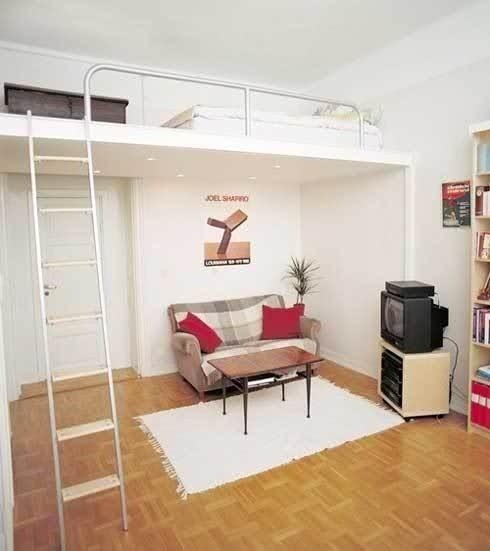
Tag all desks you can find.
[207,346,323,434]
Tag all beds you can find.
[159,105,385,151]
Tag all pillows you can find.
[177,313,223,354]
[259,304,304,340]
[316,101,378,123]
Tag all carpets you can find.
[130,376,408,502]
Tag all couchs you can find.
[166,292,325,401]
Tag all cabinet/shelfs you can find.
[4,80,131,125]
[377,343,453,423]
[464,116,490,441]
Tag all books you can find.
[471,141,490,428]
[248,372,277,387]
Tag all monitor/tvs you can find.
[380,290,448,354]
